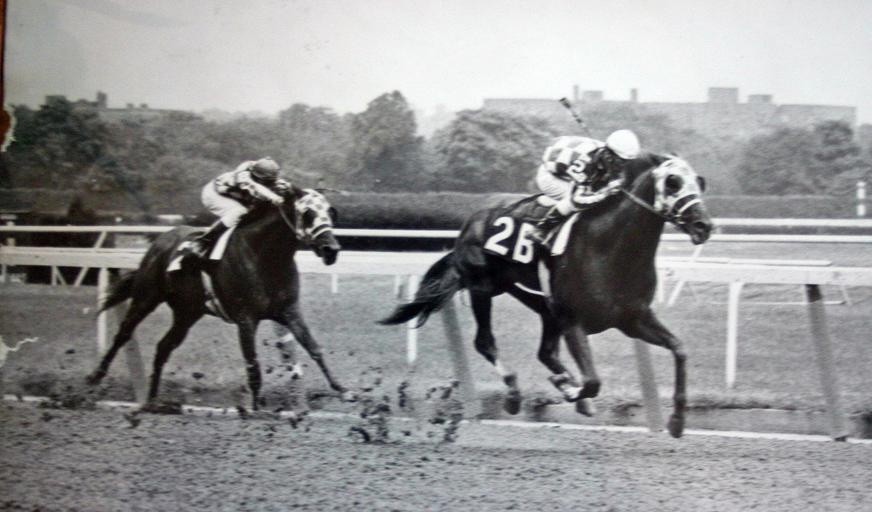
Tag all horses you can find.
[82,180,360,412]
[373,149,714,439]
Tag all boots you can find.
[525,207,569,248]
[193,221,228,257]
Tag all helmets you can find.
[605,129,641,160]
[251,156,279,181]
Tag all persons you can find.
[535,128,642,245]
[197,156,293,260]
